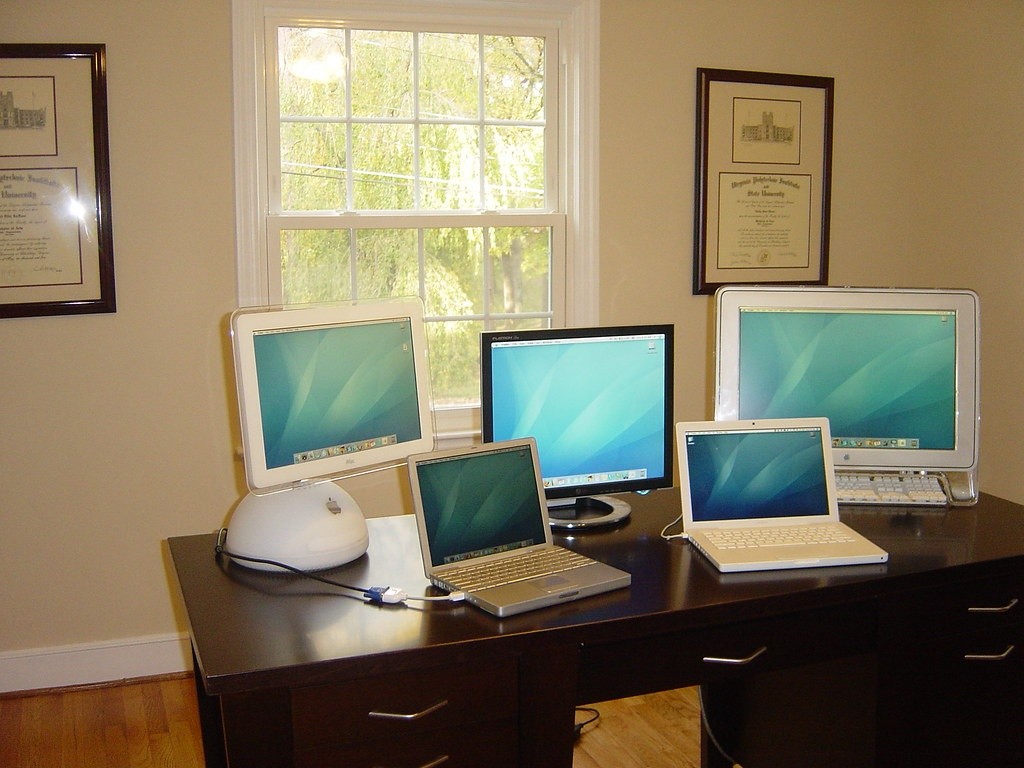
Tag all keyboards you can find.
[835,471,946,506]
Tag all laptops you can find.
[676,417,888,572]
[407,436,633,616]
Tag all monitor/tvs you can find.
[223,294,435,572]
[713,286,981,471]
[479,324,675,529]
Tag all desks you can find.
[167,485,1024,768]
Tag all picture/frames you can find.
[693,67,834,296]
[0,43,117,319]
